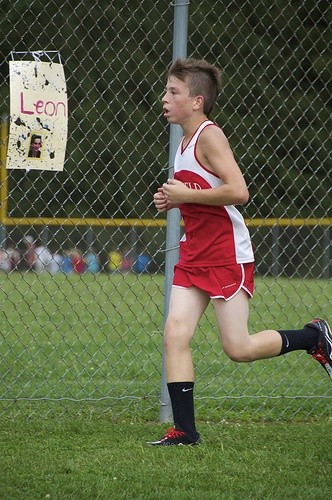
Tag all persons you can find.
[146,58,332,449]
[28,134,42,158]
[0,234,150,276]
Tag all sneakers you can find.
[305,318,332,380]
[147,428,200,447]
